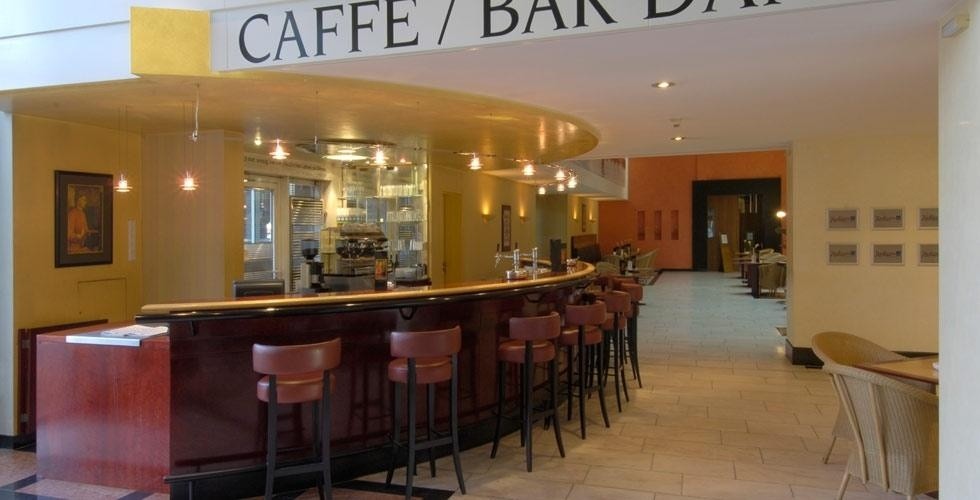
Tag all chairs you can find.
[490,275,646,475]
[249,336,352,498]
[379,324,469,499]
[734,245,787,298]
[810,329,941,498]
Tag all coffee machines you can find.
[300,238,331,293]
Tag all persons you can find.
[68,191,100,255]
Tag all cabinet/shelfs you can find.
[321,222,393,281]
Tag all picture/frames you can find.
[825,206,939,267]
[53,168,115,267]
